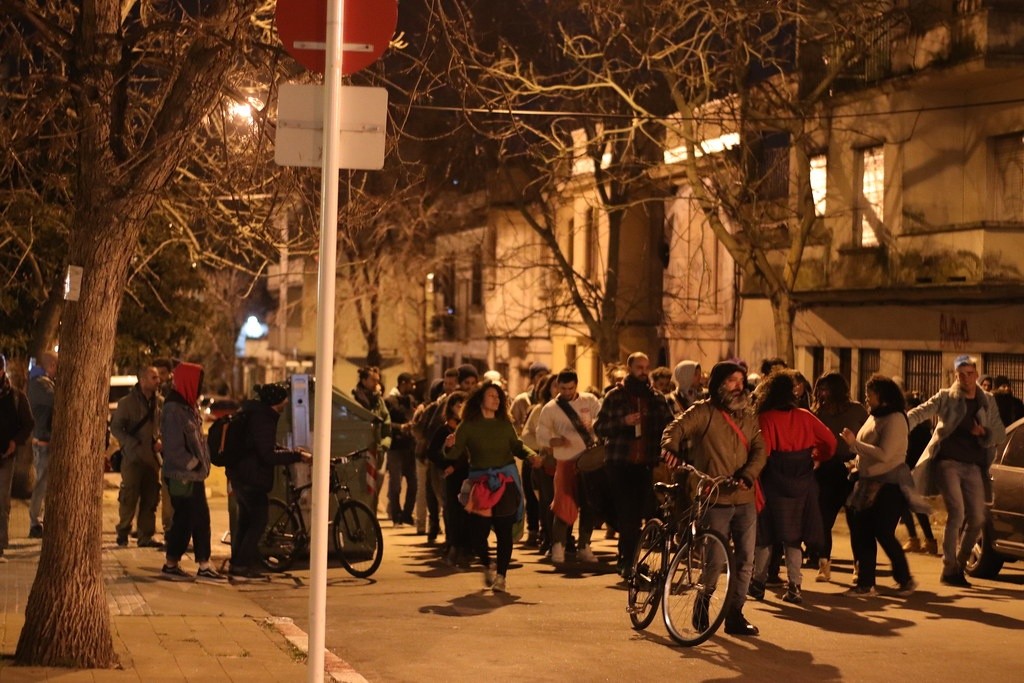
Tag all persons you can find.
[660,362,767,635]
[351,350,1024,605]
[111,358,229,582]
[0,351,58,564]
[226,383,311,583]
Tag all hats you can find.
[994,375,1009,388]
[529,362,547,377]
[955,355,974,370]
[253,383,288,406]
[458,364,478,383]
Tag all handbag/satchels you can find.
[110,450,122,472]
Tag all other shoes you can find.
[195,567,227,583]
[746,577,765,600]
[228,568,267,582]
[27,525,42,539]
[576,543,598,563]
[897,576,916,597]
[902,537,920,553]
[815,559,831,581]
[692,590,711,631]
[782,582,802,604]
[920,538,937,554]
[138,540,163,548]
[492,574,505,592]
[842,584,876,597]
[161,564,193,579]
[940,575,972,588]
[724,611,759,635]
[116,535,128,546]
[551,542,565,563]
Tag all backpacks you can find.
[207,399,258,467]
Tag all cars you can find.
[956,417,1024,580]
[108,375,139,420]
[197,394,236,422]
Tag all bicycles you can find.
[626,452,739,648]
[256,447,384,578]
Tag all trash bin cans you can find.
[266,377,384,563]
